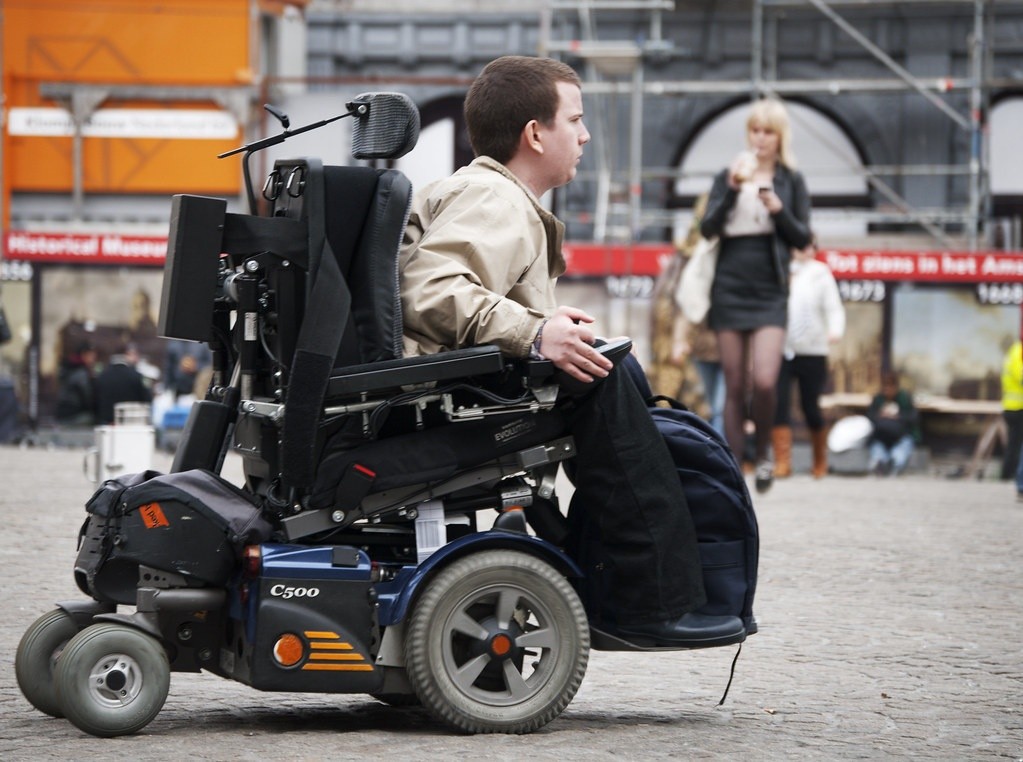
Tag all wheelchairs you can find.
[14,92,714,739]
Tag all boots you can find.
[772,425,793,479]
[809,427,828,477]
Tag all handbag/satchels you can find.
[676,234,719,324]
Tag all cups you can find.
[733,152,759,181]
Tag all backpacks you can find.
[565,394,759,628]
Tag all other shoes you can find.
[755,459,770,495]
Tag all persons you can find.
[670,96,844,491]
[864,367,921,481]
[998,341,1023,502]
[395,52,747,647]
[53,327,203,432]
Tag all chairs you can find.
[231,92,633,542]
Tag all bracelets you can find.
[534,318,548,353]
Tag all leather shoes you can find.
[607,612,746,648]
